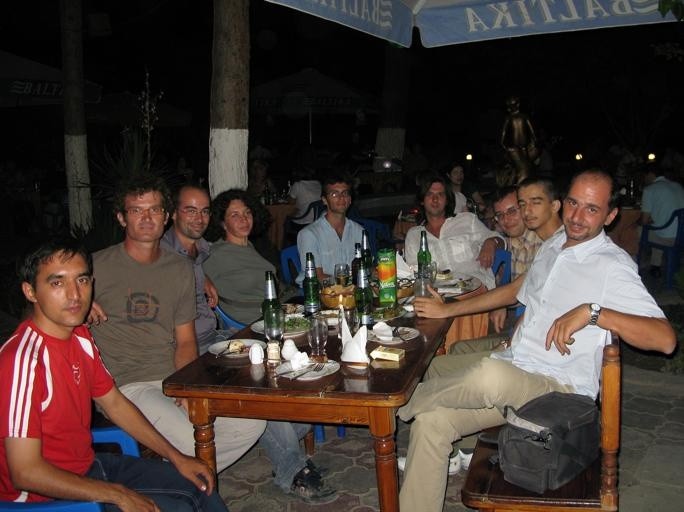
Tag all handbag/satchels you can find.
[498,392,603,492]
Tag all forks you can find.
[290,363,325,382]
[391,326,409,345]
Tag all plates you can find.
[208,338,267,359]
[367,326,420,346]
[250,313,311,339]
[274,359,341,382]
[354,308,408,323]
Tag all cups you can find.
[307,318,328,355]
[337,306,360,353]
[335,263,350,286]
[413,278,433,298]
[264,309,285,343]
[422,261,437,281]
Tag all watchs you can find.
[588,303,601,327]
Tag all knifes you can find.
[271,363,316,379]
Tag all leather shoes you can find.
[290,470,339,504]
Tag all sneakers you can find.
[396,453,461,476]
[458,451,475,470]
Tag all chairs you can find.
[1,426,145,512]
[637,206,684,296]
[459,330,627,512]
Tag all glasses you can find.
[224,210,255,218]
[175,208,213,217]
[120,206,164,217]
[492,208,520,222]
[323,189,352,199]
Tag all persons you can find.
[419,175,564,384]
[298,181,371,297]
[159,182,339,505]
[626,162,684,277]
[288,169,325,232]
[0,234,230,511]
[448,161,471,214]
[202,189,297,326]
[404,175,507,292]
[84,177,268,476]
[399,166,676,512]
[491,185,546,282]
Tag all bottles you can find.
[472,202,479,218]
[303,252,320,317]
[627,176,636,204]
[417,231,431,280]
[261,271,282,345]
[355,264,373,329]
[361,229,372,276]
[265,183,269,205]
[351,243,367,284]
[287,179,290,192]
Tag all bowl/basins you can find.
[402,305,417,319]
[320,310,339,325]
